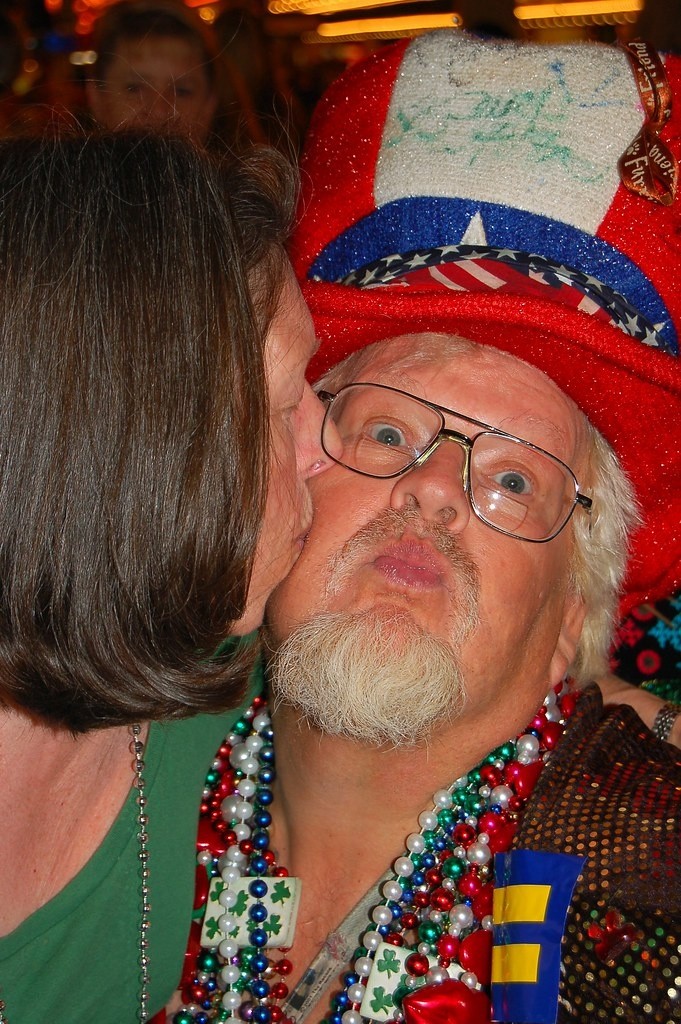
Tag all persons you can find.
[84,10,221,137]
[0,131,681,1024]
[151,27,681,1024]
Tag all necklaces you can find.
[172,673,584,1024]
[0,722,151,1024]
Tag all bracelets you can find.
[651,704,681,740]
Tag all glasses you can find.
[318,382,593,543]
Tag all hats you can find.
[283,31,681,619]
